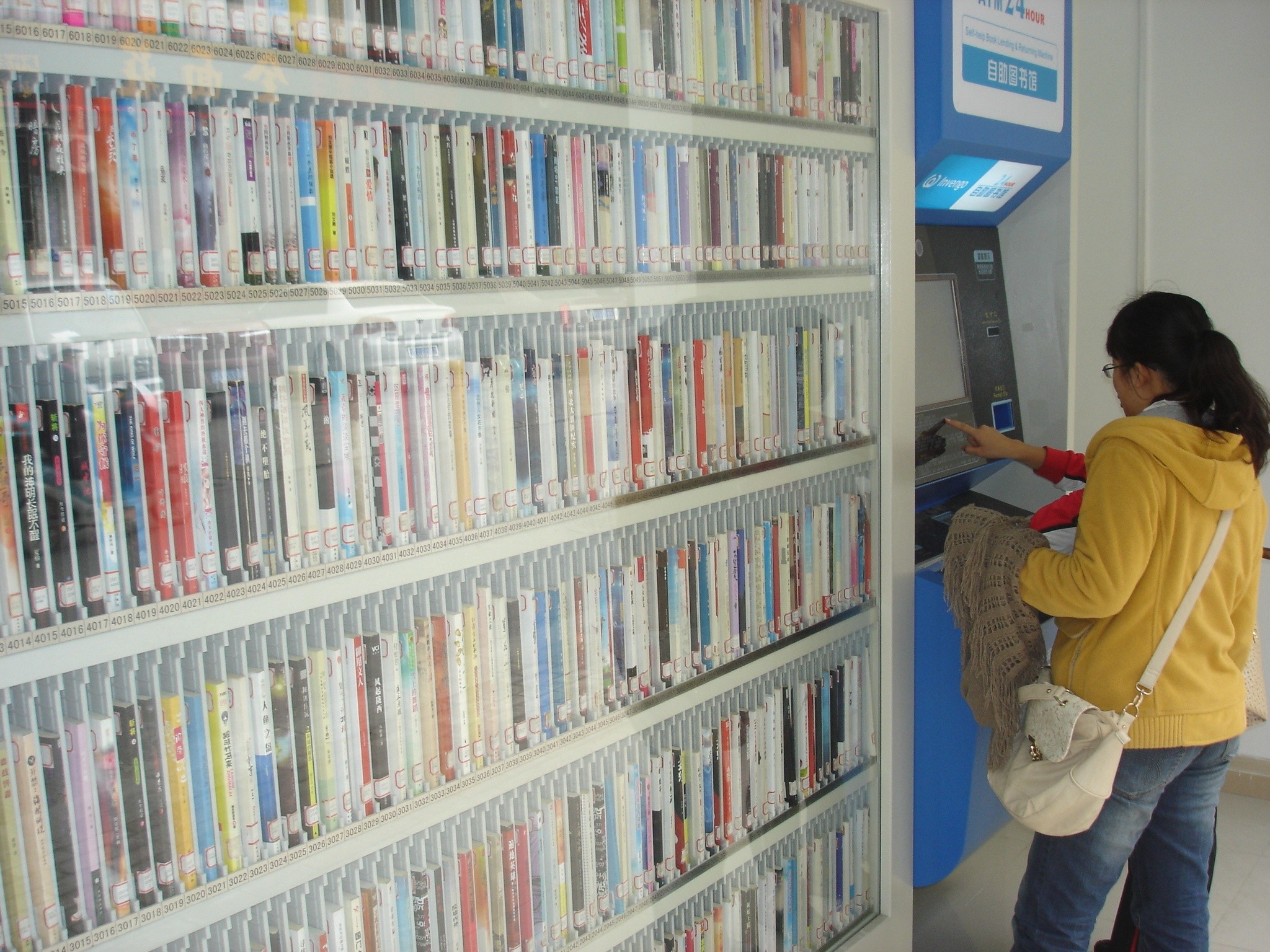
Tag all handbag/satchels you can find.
[987,666,1134,836]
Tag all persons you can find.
[944,293,1270,952]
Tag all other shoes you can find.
[1093,940,1110,952]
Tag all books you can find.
[0,0,878,294]
[0,293,878,952]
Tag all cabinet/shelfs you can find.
[0,0,914,952]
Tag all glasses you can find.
[1102,364,1155,378]
